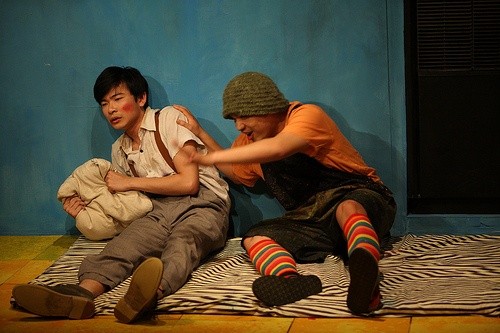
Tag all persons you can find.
[12,65,230,324]
[173,72,397,315]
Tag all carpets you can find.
[11,235,498,317]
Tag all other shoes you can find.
[347,247,380,315]
[113,256,164,323]
[252,274,323,306]
[12,284,95,320]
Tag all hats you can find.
[222,71,289,118]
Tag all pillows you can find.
[57,158,154,241]
